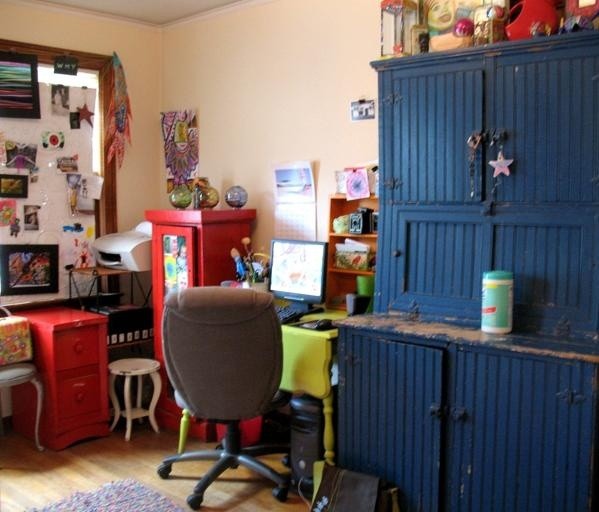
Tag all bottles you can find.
[410,23,430,57]
[479,269,515,334]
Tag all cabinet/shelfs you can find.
[68,266,154,358]
[143,203,262,446]
[326,27,597,512]
[10,305,111,453]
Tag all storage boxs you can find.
[0,315,33,369]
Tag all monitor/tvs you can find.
[269,239,329,315]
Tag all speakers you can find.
[344,293,370,317]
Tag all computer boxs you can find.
[289,394,322,494]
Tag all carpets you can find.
[24,478,187,512]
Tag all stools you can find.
[107,357,162,444]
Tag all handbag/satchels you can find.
[310,461,401,512]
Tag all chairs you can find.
[159,286,292,510]
[0,308,46,454]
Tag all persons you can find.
[420,0,473,53]
[25,212,36,225]
[164,274,189,303]
[53,89,62,108]
[176,245,186,272]
[60,86,69,107]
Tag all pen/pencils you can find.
[291,320,319,325]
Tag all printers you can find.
[90,219,153,273]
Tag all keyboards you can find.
[274,306,305,324]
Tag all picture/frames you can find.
[0,173,28,199]
[0,51,41,120]
[0,244,59,297]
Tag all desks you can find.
[173,296,349,467]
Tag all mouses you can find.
[316,318,332,328]
